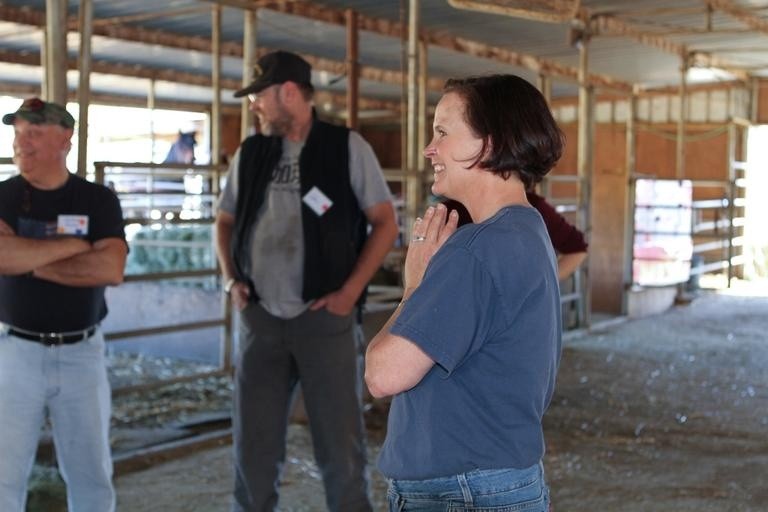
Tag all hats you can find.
[1,97,75,129]
[233,50,312,98]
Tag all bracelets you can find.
[224,276,239,294]
[23,269,33,279]
[398,298,408,306]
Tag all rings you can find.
[413,235,425,242]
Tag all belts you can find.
[0,321,104,347]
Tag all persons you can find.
[0,95,129,512]
[425,192,588,285]
[363,72,568,511]
[210,50,399,512]
